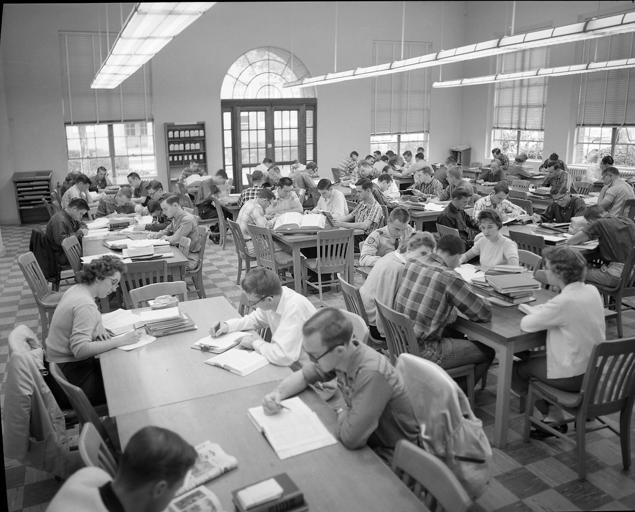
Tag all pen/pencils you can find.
[132,324,137,331]
[277,403,291,410]
[215,322,221,331]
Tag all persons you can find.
[262,306,425,470]
[44,255,143,413]
[44,425,198,512]
[538,153,568,173]
[43,158,235,271]
[357,231,438,343]
[436,155,459,190]
[471,181,527,226]
[507,153,534,181]
[511,243,606,441]
[436,186,483,265]
[590,167,635,217]
[459,209,520,267]
[582,155,614,182]
[358,147,435,206]
[485,148,510,170]
[236,152,357,294]
[541,161,578,193]
[532,182,587,223]
[435,165,475,208]
[208,267,338,402]
[357,206,415,267]
[358,206,386,252]
[391,233,496,397]
[473,159,507,187]
[565,204,634,287]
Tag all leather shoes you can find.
[528,420,568,441]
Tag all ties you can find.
[394,239,398,250]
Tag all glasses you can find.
[553,195,566,203]
[149,189,157,198]
[249,294,273,307]
[105,277,119,289]
[306,344,344,363]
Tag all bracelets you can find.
[337,404,351,414]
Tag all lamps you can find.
[88,1,217,92]
[283,1,634,88]
[432,2,634,87]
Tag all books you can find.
[230,472,308,512]
[403,201,425,211]
[470,264,542,308]
[101,308,157,350]
[105,185,121,196]
[535,222,571,246]
[140,306,199,337]
[84,213,139,239]
[104,232,174,262]
[203,343,270,377]
[161,484,223,512]
[569,216,589,235]
[511,217,534,226]
[248,395,338,460]
[172,439,238,496]
[501,215,525,226]
[274,209,339,234]
